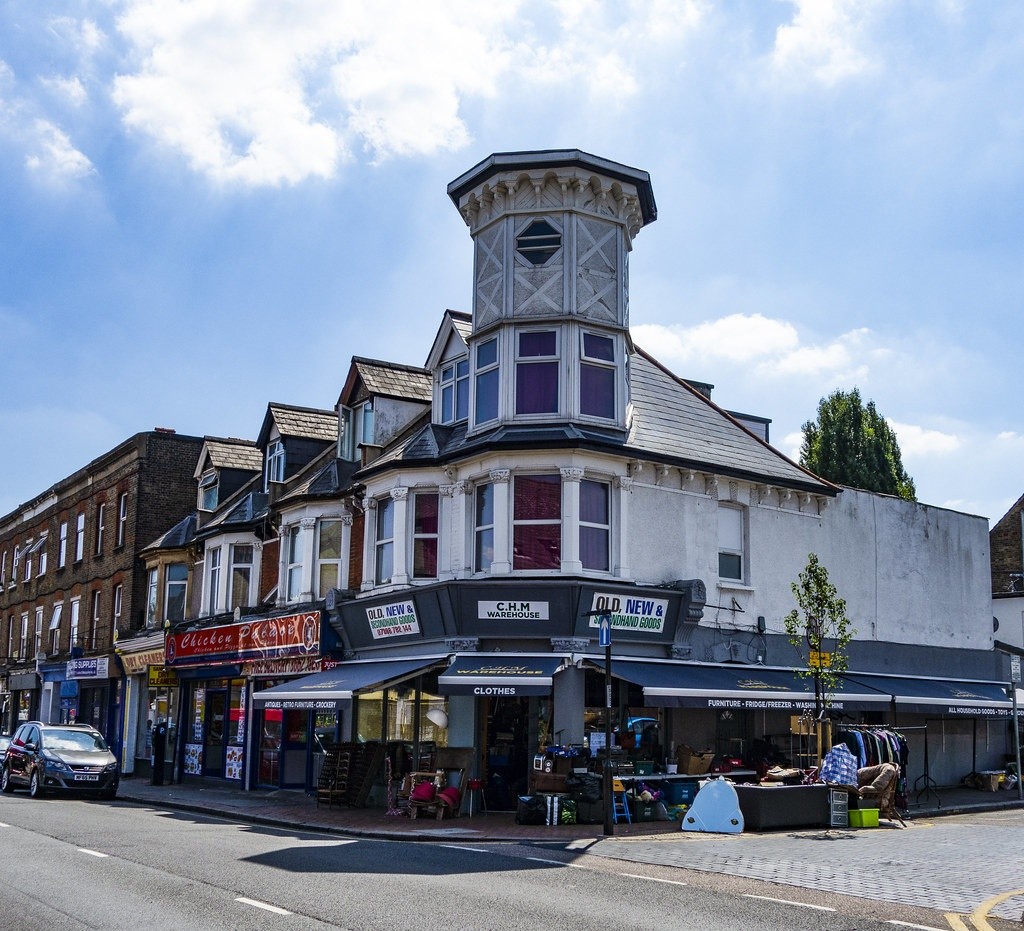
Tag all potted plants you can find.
[538,720,548,753]
[847,808,880,828]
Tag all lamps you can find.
[426,710,448,730]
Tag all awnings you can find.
[590,657,1024,716]
[438,656,563,696]
[251,658,443,711]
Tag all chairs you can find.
[409,747,476,819]
[317,749,351,810]
[849,762,907,829]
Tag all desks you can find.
[611,779,631,825]
[733,783,832,831]
[589,767,757,782]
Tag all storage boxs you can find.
[981,773,1000,792]
[667,781,697,804]
[636,761,654,775]
[627,796,658,820]
[679,752,717,775]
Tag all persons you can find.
[146,720,153,757]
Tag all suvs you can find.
[0,720,120,799]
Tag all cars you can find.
[613,716,659,752]
[227,735,280,780]
